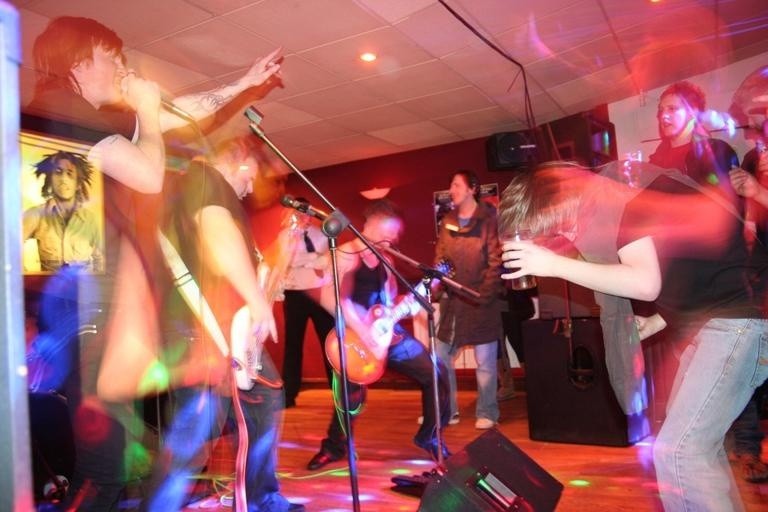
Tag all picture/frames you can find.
[18,132,107,277]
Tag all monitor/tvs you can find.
[548,116,618,172]
[485,132,531,171]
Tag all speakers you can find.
[523,315,653,446]
[417,426,564,512]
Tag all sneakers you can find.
[472,418,499,431]
[415,410,461,425]
[738,450,767,483]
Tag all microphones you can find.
[279,194,329,222]
[120,76,195,122]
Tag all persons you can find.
[1,14,767,512]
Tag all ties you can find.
[303,230,319,255]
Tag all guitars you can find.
[326,253,455,386]
[220,218,303,393]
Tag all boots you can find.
[492,352,517,403]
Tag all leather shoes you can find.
[412,433,452,462]
[306,442,347,469]
